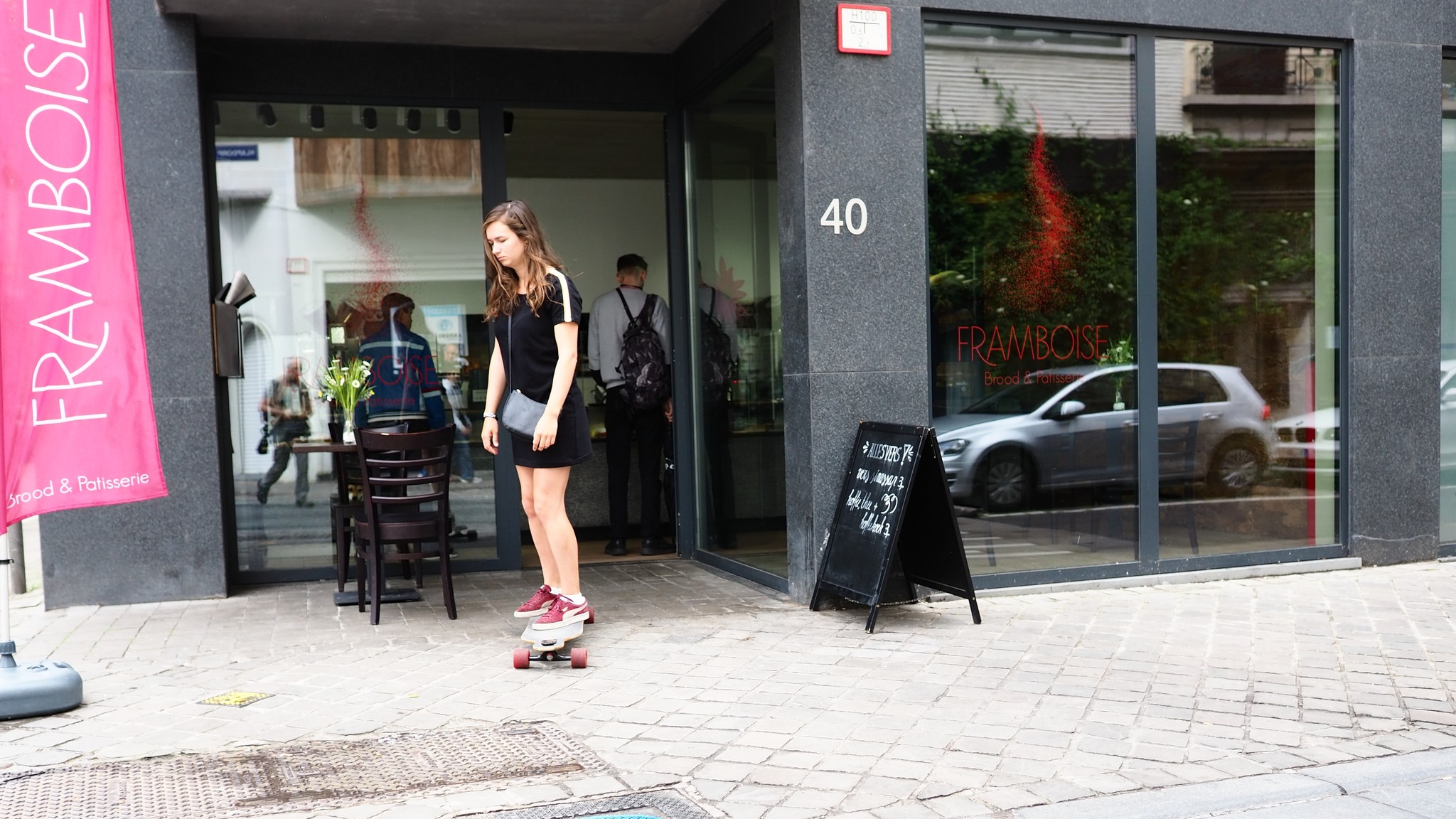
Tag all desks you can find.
[290,433,461,608]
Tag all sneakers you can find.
[513,584,557,618]
[532,594,590,631]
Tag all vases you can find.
[341,408,358,447]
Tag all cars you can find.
[1273,360,1456,491]
[934,364,1277,514]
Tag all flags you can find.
[0,0,166,535]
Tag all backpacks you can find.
[699,286,734,391]
[616,287,667,409]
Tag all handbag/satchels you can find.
[503,389,562,443]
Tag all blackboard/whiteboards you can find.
[818,421,976,605]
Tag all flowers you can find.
[313,353,377,433]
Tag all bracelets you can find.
[483,412,499,421]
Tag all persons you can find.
[360,295,460,540]
[697,259,744,553]
[480,199,594,641]
[257,356,317,508]
[589,253,674,557]
[417,341,483,486]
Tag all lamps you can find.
[502,109,514,137]
[213,100,222,128]
[307,103,325,132]
[257,102,279,129]
[444,107,462,134]
[359,106,379,132]
[403,108,422,134]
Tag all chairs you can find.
[351,420,462,626]
[329,418,421,591]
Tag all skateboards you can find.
[512,605,595,669]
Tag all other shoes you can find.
[641,540,677,555]
[256,478,269,504]
[296,498,315,508]
[605,540,627,556]
[462,476,482,484]
[709,537,741,550]
[421,548,459,560]
